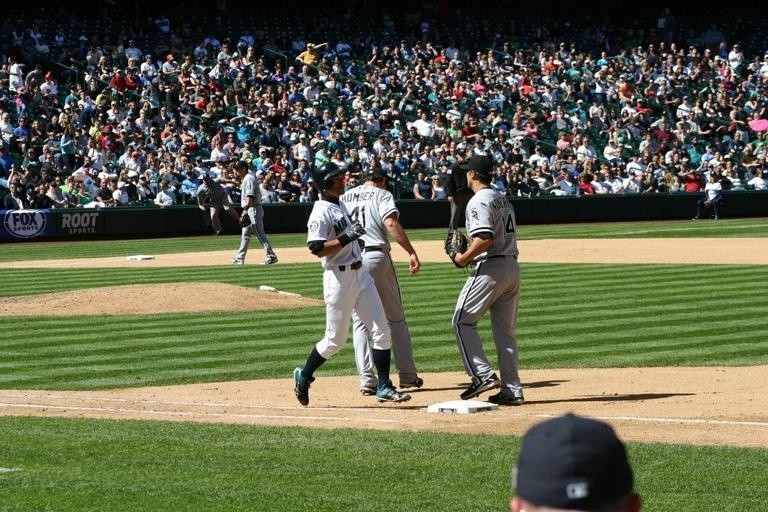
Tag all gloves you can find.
[346,220,366,240]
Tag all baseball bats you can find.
[259,286,302,298]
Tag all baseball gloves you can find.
[239,213,251,228]
[445,228,467,267]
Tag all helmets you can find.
[315,162,347,189]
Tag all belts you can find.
[339,260,362,271]
[365,246,381,252]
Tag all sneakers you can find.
[293,366,524,406]
[231,257,244,264]
[264,257,278,264]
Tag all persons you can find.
[451,156,526,406]
[230,160,278,264]
[340,167,420,395]
[505,411,641,512]
[292,163,411,405]
[1,3,768,227]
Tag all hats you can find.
[234,161,248,168]
[517,413,632,507]
[363,168,392,180]
[460,155,492,170]
[457,148,466,155]
